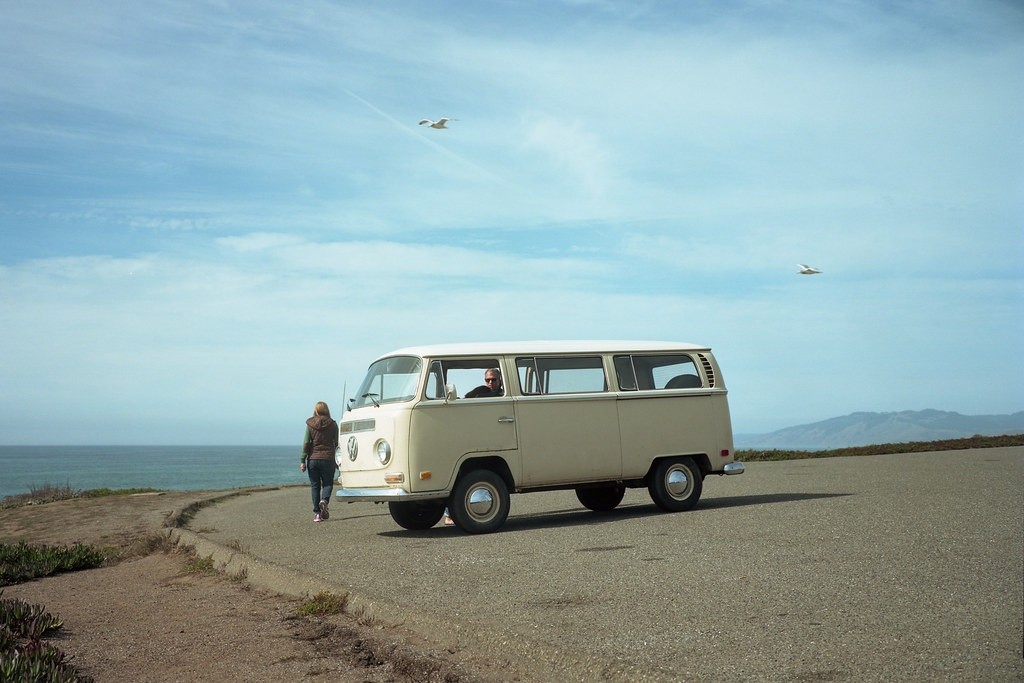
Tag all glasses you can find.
[485,378,497,382]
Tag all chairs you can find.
[465,386,494,398]
[665,374,701,389]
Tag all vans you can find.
[334,338,747,531]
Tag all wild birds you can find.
[796,264,824,276]
[419,118,458,130]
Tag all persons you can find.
[465,368,504,398]
[300,400,339,522]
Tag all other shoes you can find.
[313,514,321,522]
[319,499,330,520]
